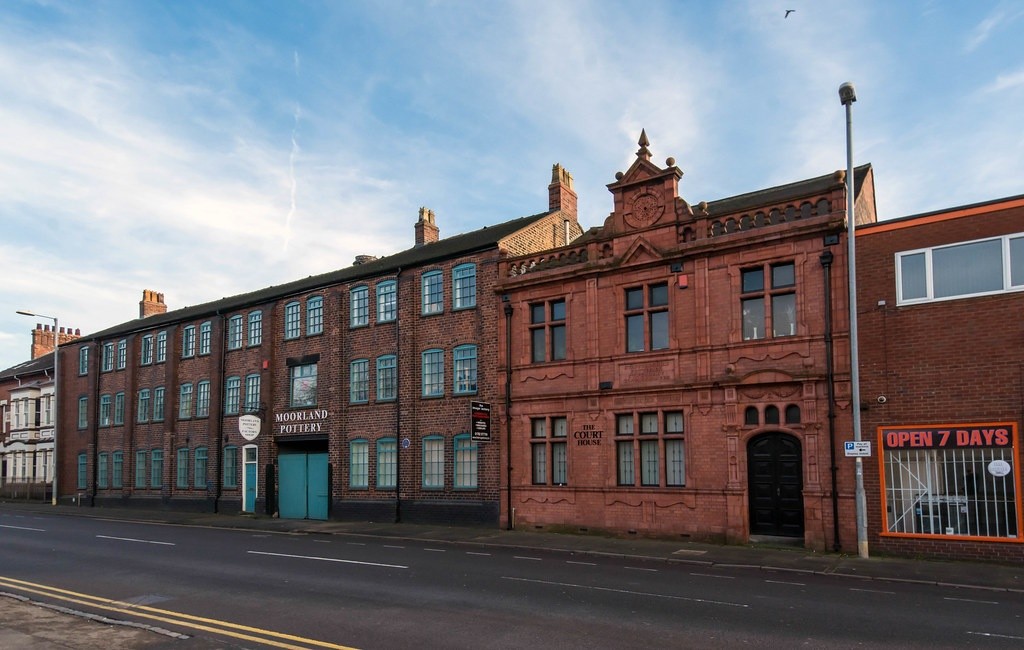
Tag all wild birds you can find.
[785,9,795,19]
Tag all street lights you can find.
[837,81,870,558]
[16,310,57,505]
